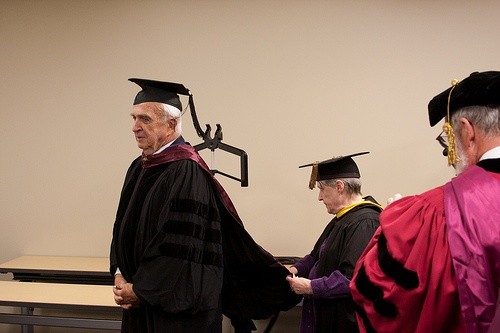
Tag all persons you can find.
[349,71,500,333]
[109,78,303,333]
[286,152,384,333]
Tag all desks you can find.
[0,281,124,330]
[0,254,115,333]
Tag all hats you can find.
[428,70,500,166]
[299,152,369,190]
[128,78,204,138]
